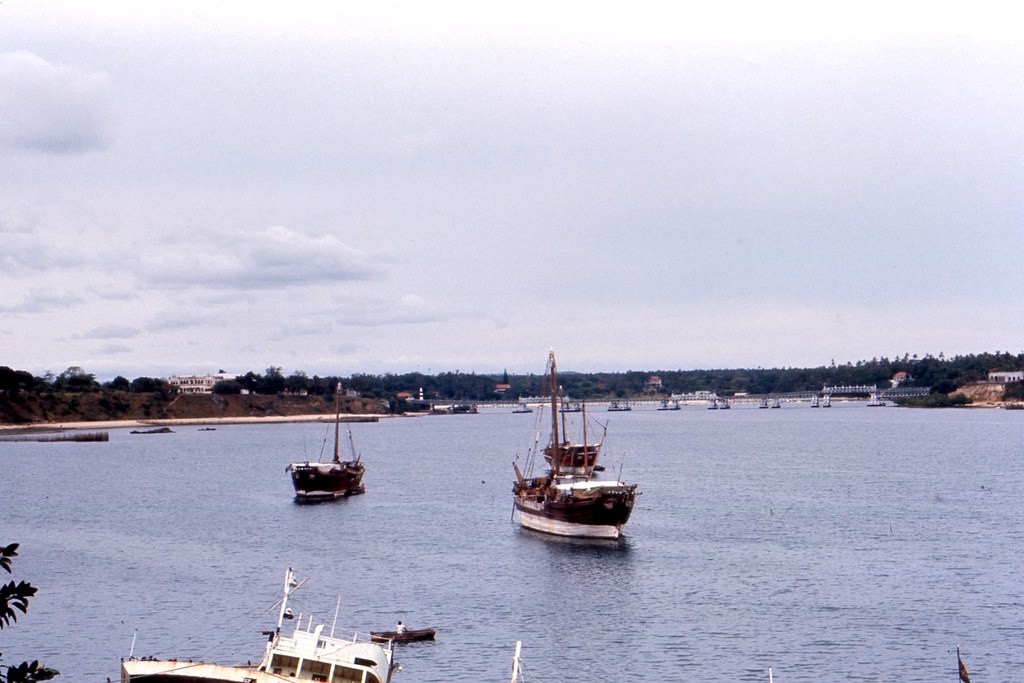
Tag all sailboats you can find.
[285,380,364,507]
[510,349,638,544]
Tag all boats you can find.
[759,399,768,408]
[810,399,819,407]
[558,405,581,413]
[368,626,437,645]
[607,406,632,412]
[772,401,780,408]
[512,403,533,414]
[707,402,719,409]
[822,401,831,407]
[719,402,730,409]
[657,404,680,410]
[866,401,886,407]
[119,568,402,683]
